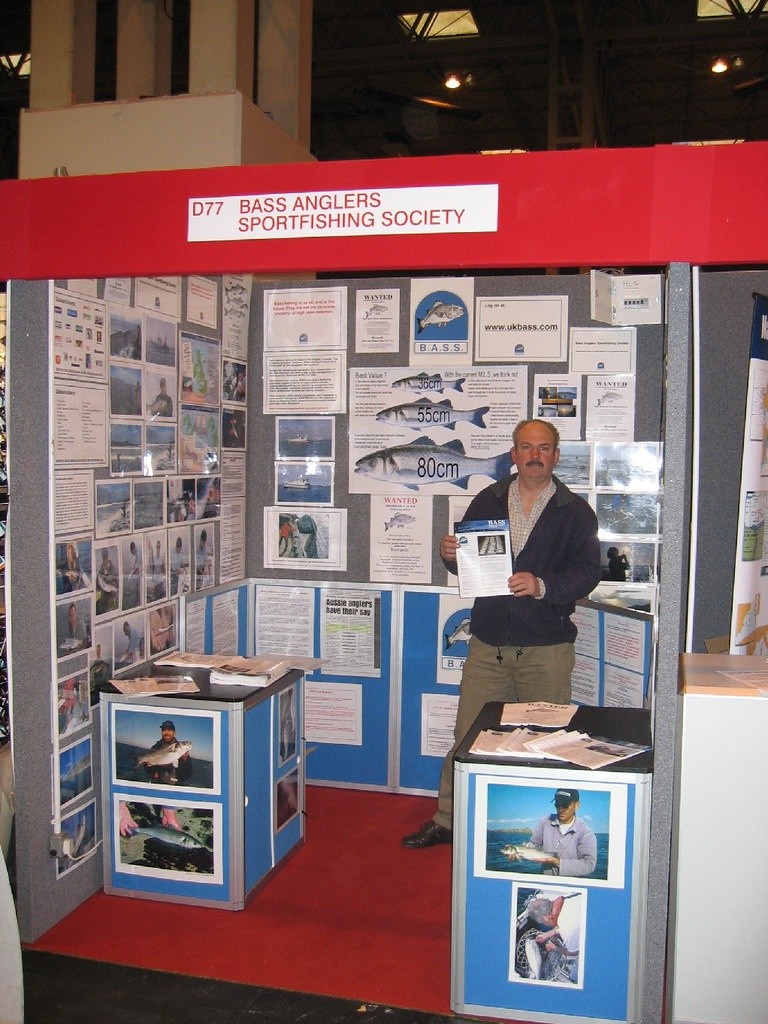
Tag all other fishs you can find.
[499,844,557,865]
[96,574,119,594]
[390,372,466,395]
[354,435,517,492]
[73,814,87,857]
[134,740,192,771]
[384,511,415,531]
[131,826,203,850]
[146,399,168,415]
[154,624,174,636]
[524,936,542,979]
[376,397,490,431]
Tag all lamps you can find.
[710,51,748,73]
[443,67,478,89]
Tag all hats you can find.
[160,378,166,385]
[160,720,175,730]
[551,788,579,803]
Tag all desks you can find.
[450,700,654,1024]
[99,667,309,911]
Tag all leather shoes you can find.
[401,820,451,848]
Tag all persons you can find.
[607,546,631,581]
[508,788,598,879]
[175,498,188,521]
[529,891,582,980]
[129,541,141,606]
[280,519,296,557]
[170,538,185,595]
[88,643,105,682]
[195,530,210,589]
[144,719,193,785]
[147,540,164,596]
[59,542,84,593]
[398,415,603,849]
[62,603,88,649]
[119,801,182,836]
[121,620,141,662]
[154,377,174,417]
[97,548,118,613]
[235,363,246,401]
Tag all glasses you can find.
[554,803,575,809]
